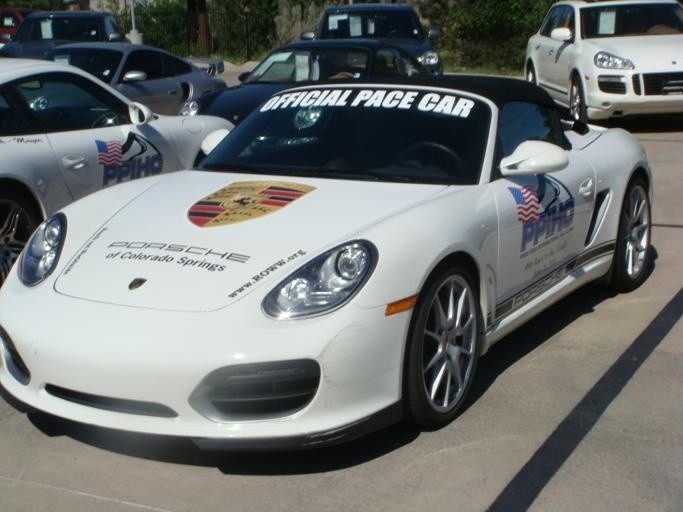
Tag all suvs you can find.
[298,3,447,77]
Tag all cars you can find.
[38,42,228,118]
[519,1,682,127]
[0,10,132,69]
[184,37,427,130]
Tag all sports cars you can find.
[0,56,239,286]
[0,67,658,459]
